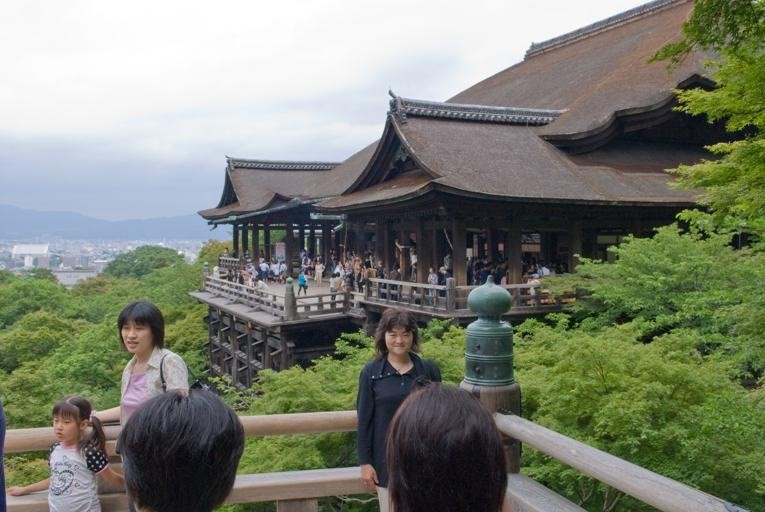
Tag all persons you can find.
[383,381,509,511]
[110,386,246,511]
[327,249,400,304]
[427,266,451,297]
[467,254,508,285]
[3,394,125,511]
[301,248,325,286]
[409,261,417,294]
[245,250,286,298]
[298,271,307,295]
[354,306,444,511]
[223,248,254,295]
[85,300,191,512]
[521,259,567,303]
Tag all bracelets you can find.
[91,409,96,415]
